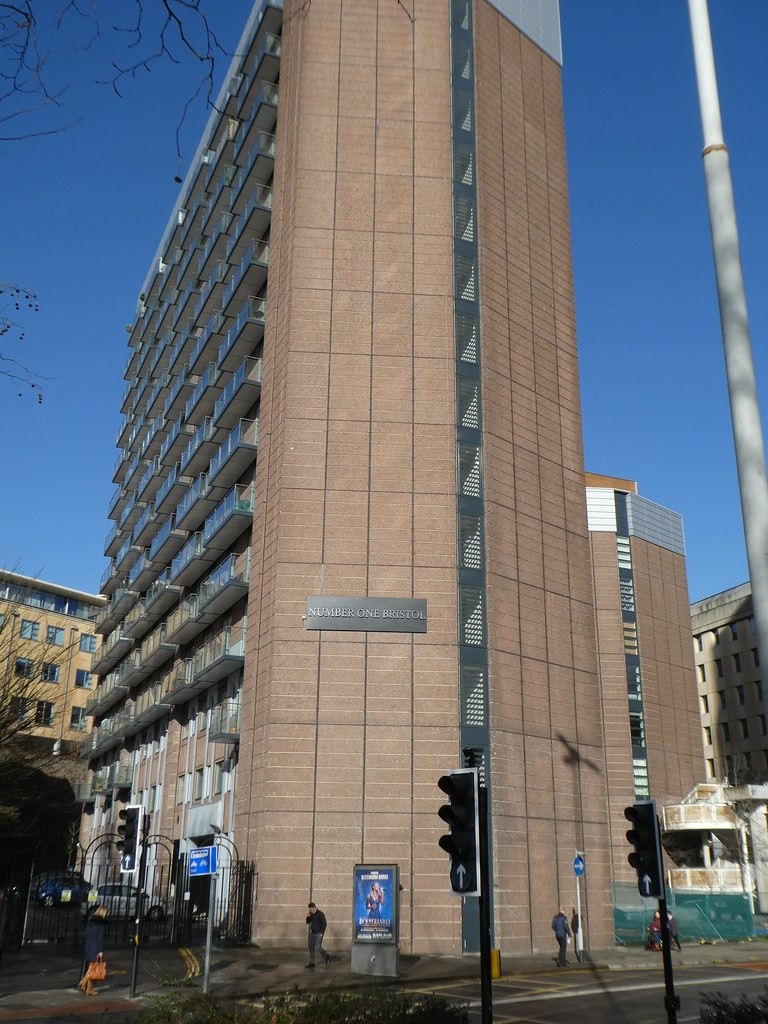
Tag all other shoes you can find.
[304,963,316,968]
[325,954,330,965]
[678,947,682,952]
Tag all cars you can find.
[3,869,83,902]
[86,882,165,923]
[36,876,93,909]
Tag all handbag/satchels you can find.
[86,954,107,980]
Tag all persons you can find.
[79,906,109,996]
[552,906,571,968]
[305,903,330,968]
[644,910,682,952]
[366,882,384,919]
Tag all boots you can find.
[86,979,98,996]
[79,975,87,993]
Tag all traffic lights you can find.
[115,802,143,873]
[622,800,665,900]
[437,766,483,898]
